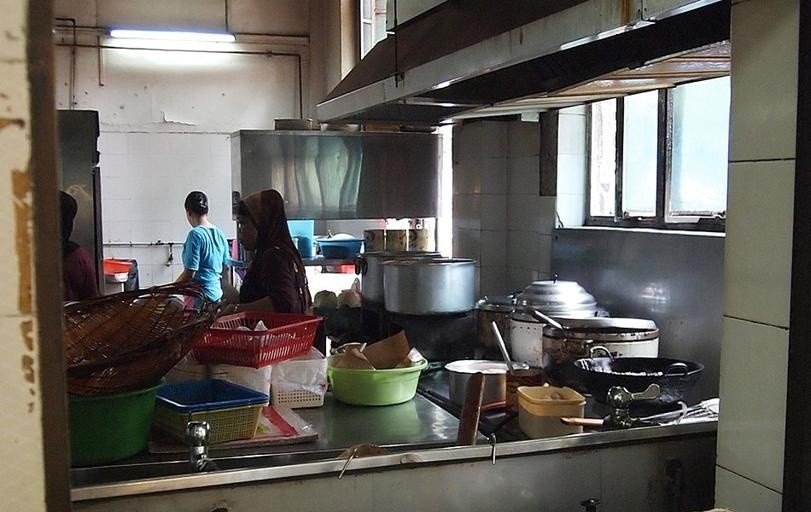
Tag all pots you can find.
[509,279,609,369]
[359,251,445,306]
[381,256,477,316]
[573,344,705,410]
[540,316,659,388]
[475,291,521,351]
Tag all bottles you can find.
[232,239,238,259]
[363,228,429,252]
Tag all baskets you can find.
[65,279,326,447]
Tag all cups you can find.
[506,368,545,414]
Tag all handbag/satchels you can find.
[221,262,240,301]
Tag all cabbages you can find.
[338,289,362,307]
[314,290,337,307]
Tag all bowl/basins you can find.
[317,239,365,260]
[326,353,429,408]
[444,359,509,405]
[103,258,133,274]
[67,375,168,466]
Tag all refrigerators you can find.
[53,109,103,297]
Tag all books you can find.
[148,403,319,455]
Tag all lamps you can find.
[109,28,236,44]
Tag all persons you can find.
[174,191,231,317]
[214,189,313,315]
[58,188,100,300]
